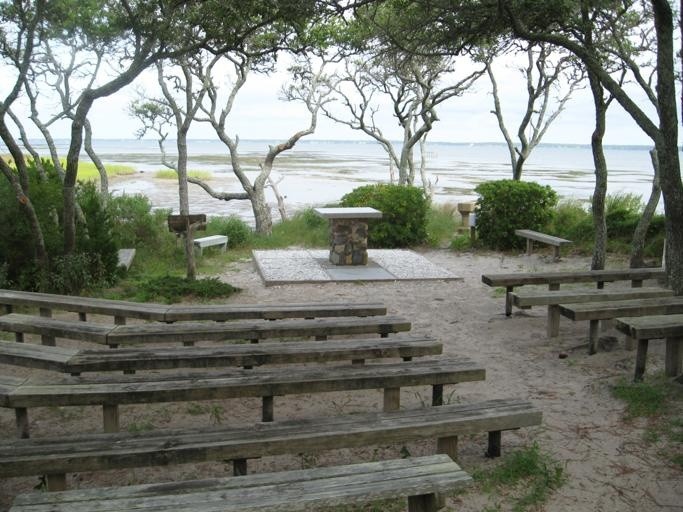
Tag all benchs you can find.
[515,230,573,263]
[115,248,136,271]
[482,266,665,316]
[559,295,683,355]
[508,286,675,337]
[616,314,683,381]
[193,234,229,256]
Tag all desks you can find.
[314,206,383,266]
